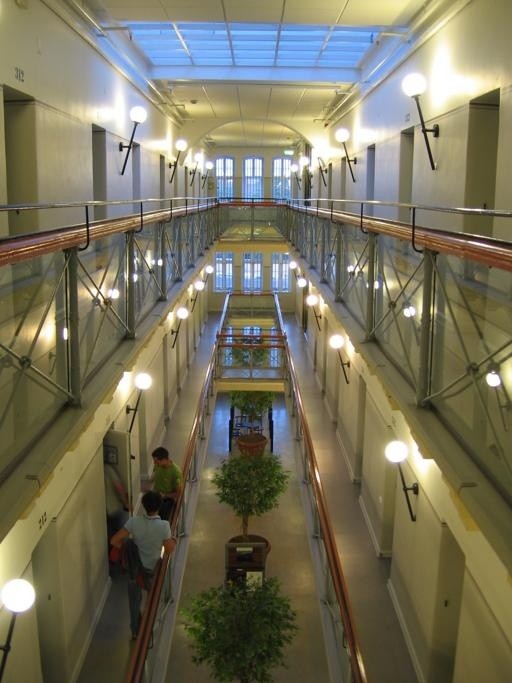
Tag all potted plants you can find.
[211,446,290,589]
[228,387,276,460]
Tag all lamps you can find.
[326,332,352,387]
[188,152,204,188]
[399,69,444,175]
[115,101,147,173]
[383,436,424,523]
[1,573,36,680]
[122,369,154,432]
[287,258,326,334]
[165,137,187,183]
[334,127,359,182]
[201,162,215,188]
[164,264,214,350]
[285,143,329,191]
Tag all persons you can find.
[102,462,130,578]
[142,446,183,521]
[110,491,178,640]
